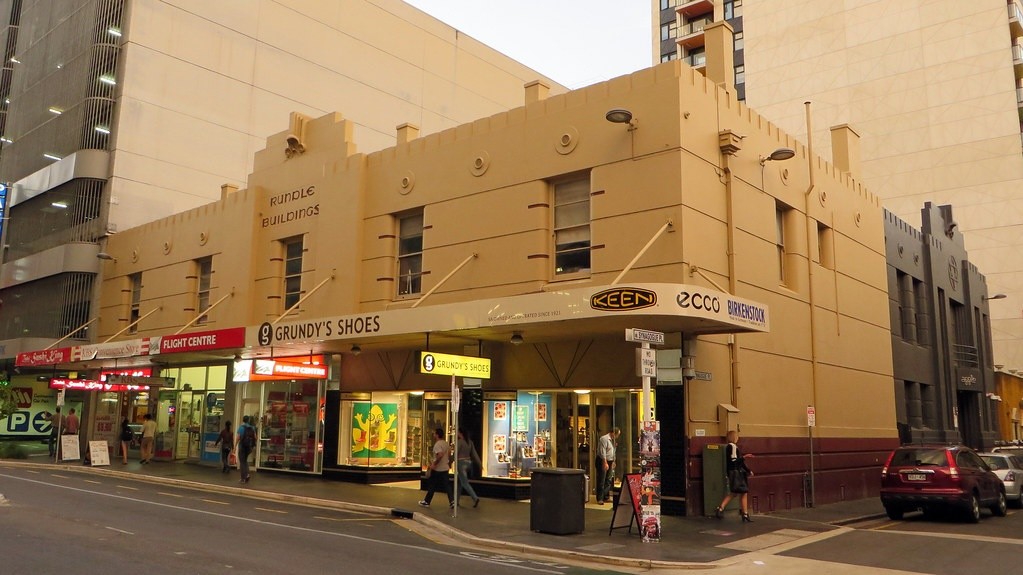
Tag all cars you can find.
[879,444,1022,524]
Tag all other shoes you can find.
[450,501,455,508]
[605,497,613,501]
[598,500,604,505]
[146,460,150,463]
[240,475,250,483]
[473,497,479,508]
[419,500,430,507]
[140,459,146,464]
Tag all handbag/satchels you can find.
[227,452,237,466]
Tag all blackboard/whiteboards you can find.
[83,441,110,466]
[59,435,80,460]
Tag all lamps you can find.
[96,253,118,263]
[759,147,795,166]
[350,344,362,356]
[511,335,524,346]
[606,109,638,132]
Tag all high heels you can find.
[716,509,724,520]
[742,513,754,522]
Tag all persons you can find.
[214,420,234,475]
[448,426,482,508]
[232,416,258,484]
[418,428,454,508]
[715,430,756,522]
[139,414,159,465]
[116,419,136,464]
[596,427,621,505]
[45,406,66,457]
[62,408,79,435]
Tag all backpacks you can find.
[122,427,133,442]
[242,425,258,452]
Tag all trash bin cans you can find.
[530,467,587,533]
